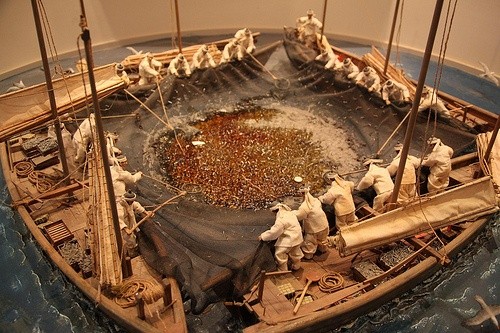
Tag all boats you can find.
[284,0,499,132]
[242,172,491,333]
[1,0,187,332]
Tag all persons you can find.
[417,85,451,119]
[423,136,454,192]
[166,53,192,78]
[317,48,360,82]
[355,66,380,93]
[386,141,422,203]
[138,52,163,85]
[296,8,323,36]
[115,62,130,87]
[192,45,216,71]
[74,113,154,258]
[259,201,305,274]
[219,27,255,64]
[382,79,410,106]
[357,157,394,211]
[317,171,358,231]
[290,184,329,260]
